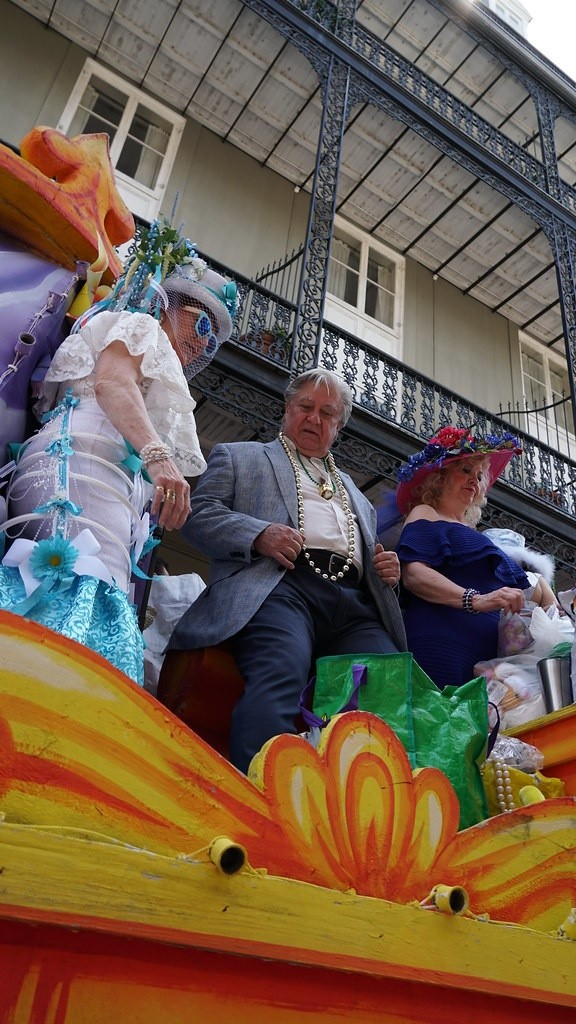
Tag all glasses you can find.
[180,305,218,358]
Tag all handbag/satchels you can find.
[297,652,500,831]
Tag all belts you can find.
[305,549,362,589]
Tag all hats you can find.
[160,262,240,347]
[393,426,521,519]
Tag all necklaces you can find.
[278,432,355,580]
[295,449,336,500]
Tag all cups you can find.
[536,656,573,714]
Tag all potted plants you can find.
[239,322,293,364]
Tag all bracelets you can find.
[463,588,480,614]
[139,441,175,467]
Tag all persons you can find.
[396,439,556,687]
[10,251,221,690]
[181,369,412,774]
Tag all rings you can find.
[166,488,176,503]
[157,486,166,492]
